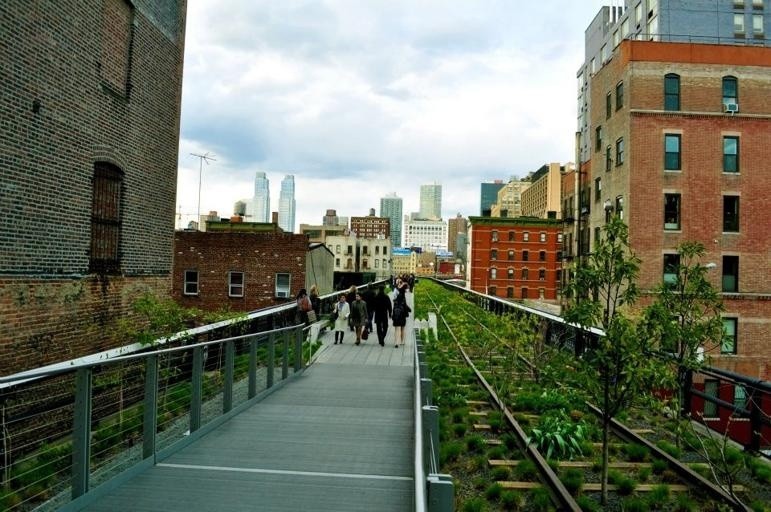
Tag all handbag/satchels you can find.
[361,325,369,340]
[301,298,312,313]
[331,303,338,322]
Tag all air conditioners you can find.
[724,102,739,112]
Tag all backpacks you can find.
[393,306,404,322]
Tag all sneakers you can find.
[334,341,343,344]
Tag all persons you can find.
[347,286,359,332]
[389,270,420,292]
[296,289,312,334]
[331,293,352,344]
[392,294,413,348]
[349,293,368,346]
[363,282,377,334]
[373,284,392,347]
[309,284,323,321]
